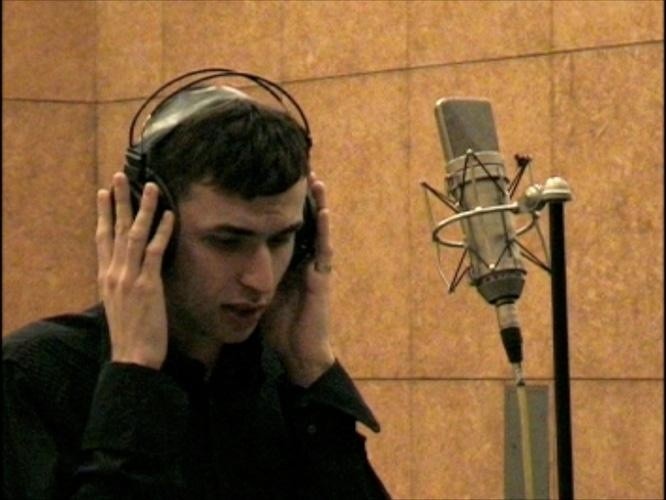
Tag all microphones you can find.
[432,95,528,304]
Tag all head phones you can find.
[109,66,322,277]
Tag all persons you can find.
[1,82,391,500]
